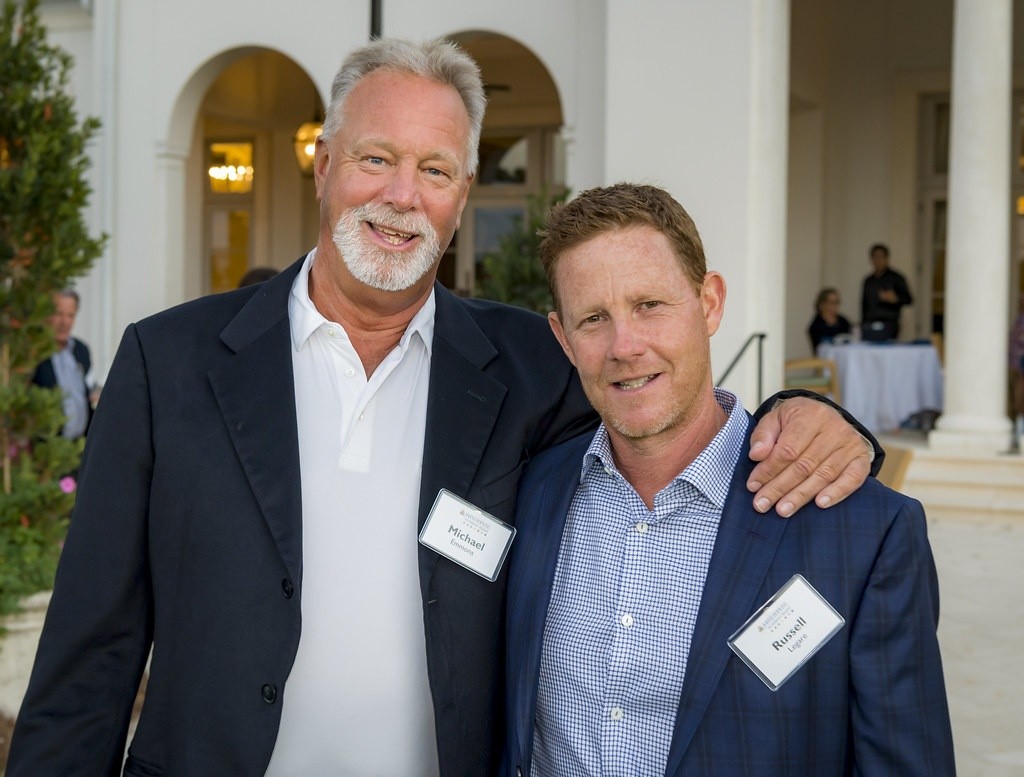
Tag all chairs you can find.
[785,356,842,411]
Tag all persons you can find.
[806,287,852,359]
[856,243,915,344]
[30,288,96,488]
[502,183,957,776]
[4,35,888,777]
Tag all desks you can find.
[817,340,947,435]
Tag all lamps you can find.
[293,85,327,176]
